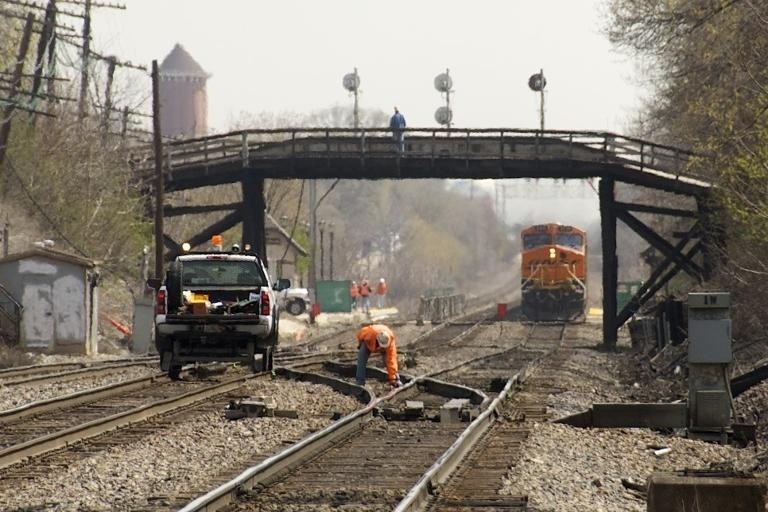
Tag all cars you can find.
[267,273,310,318]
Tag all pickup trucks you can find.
[145,233,293,380]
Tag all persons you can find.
[356,324,404,388]
[350,280,360,308]
[389,106,406,157]
[358,280,371,314]
[375,278,387,309]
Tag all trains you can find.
[518,222,591,329]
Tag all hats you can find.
[377,332,390,349]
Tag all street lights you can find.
[278,214,335,281]
